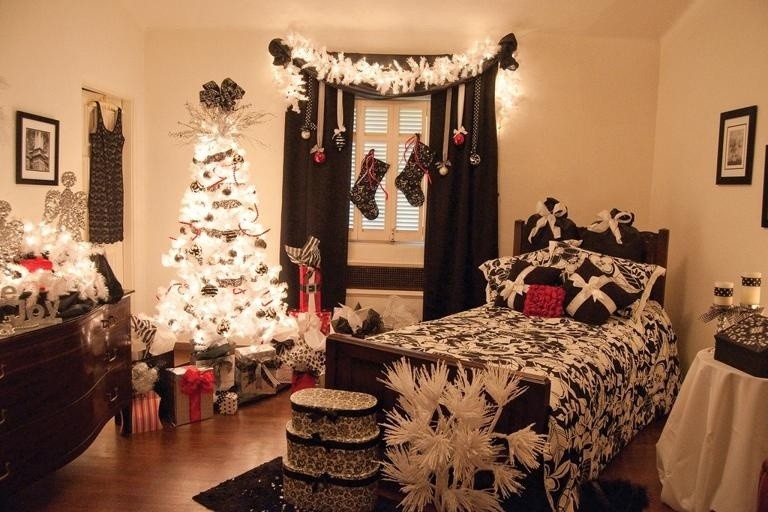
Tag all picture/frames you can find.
[16,111,59,185]
[716,106,757,184]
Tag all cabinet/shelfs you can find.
[0,289,135,503]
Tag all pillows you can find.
[478,239,667,337]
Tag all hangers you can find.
[87,94,124,114]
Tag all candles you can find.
[741,272,762,304]
[714,282,734,306]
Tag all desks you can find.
[656,346,768,512]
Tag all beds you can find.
[326,213,679,510]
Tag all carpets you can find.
[192,456,650,511]
